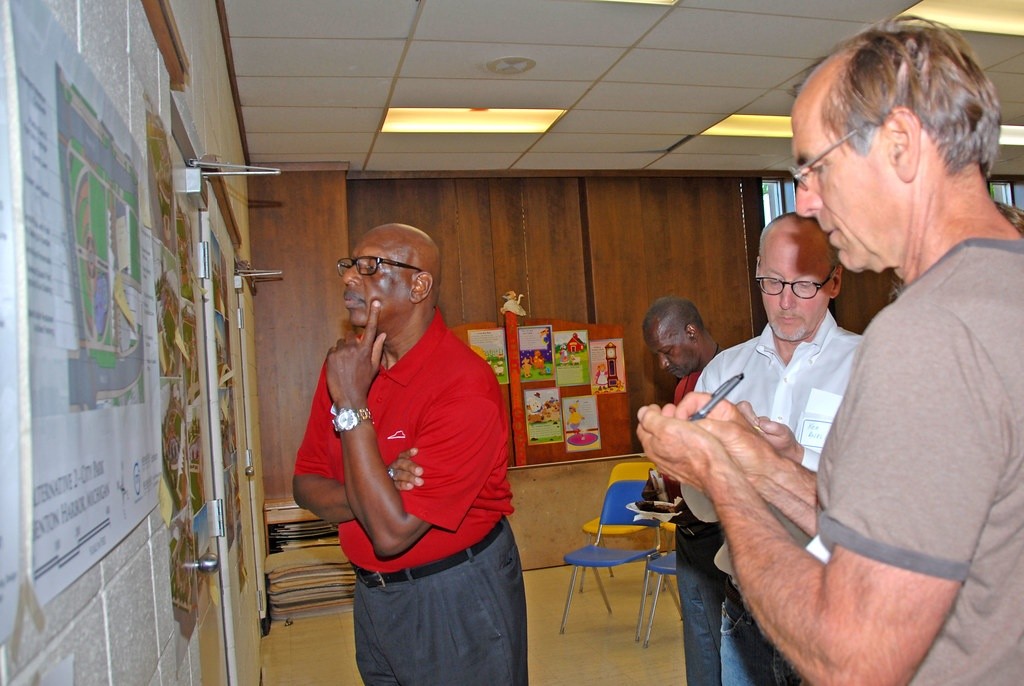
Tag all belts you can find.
[348,515,504,589]
[675,524,721,540]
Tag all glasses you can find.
[754,256,838,299]
[337,256,421,284]
[787,122,868,192]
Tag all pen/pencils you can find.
[688,373,746,421]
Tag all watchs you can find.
[331,407,372,431]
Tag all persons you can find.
[635,12,1024,686]
[292,222,528,686]
[642,201,1024,686]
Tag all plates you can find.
[626,500,682,516]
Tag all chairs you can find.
[559,462,682,648]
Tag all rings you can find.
[387,467,396,478]
